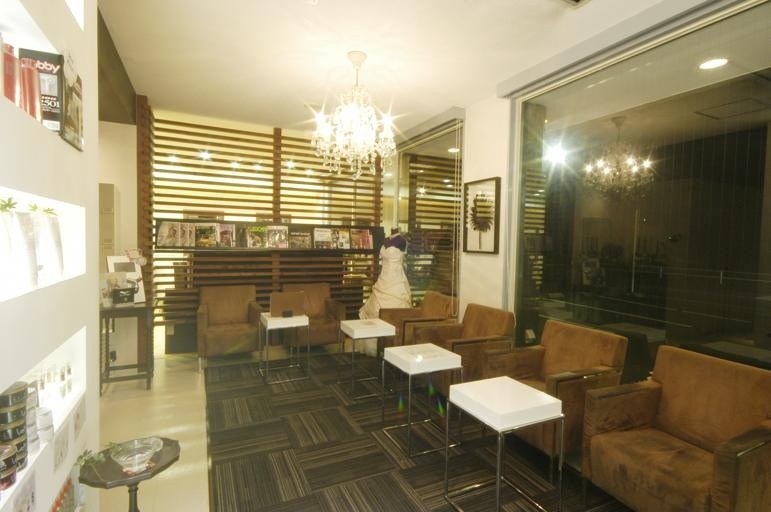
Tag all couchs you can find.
[378,292,457,354]
[612,329,698,384]
[404,303,515,429]
[581,344,768,510]
[480,318,628,487]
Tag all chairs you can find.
[280,282,346,367]
[594,325,705,384]
[194,284,266,372]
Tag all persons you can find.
[355,225,412,358]
[427,233,457,296]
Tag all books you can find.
[411,228,545,253]
[156,220,376,249]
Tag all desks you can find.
[99,298,158,397]
[335,318,398,402]
[380,343,464,457]
[444,376,564,511]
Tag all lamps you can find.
[309,49,397,184]
[584,113,657,201]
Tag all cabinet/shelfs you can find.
[0,0,100,512]
[99,183,121,290]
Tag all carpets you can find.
[204,351,633,512]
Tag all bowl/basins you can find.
[110,435,165,474]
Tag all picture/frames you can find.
[462,176,501,255]
